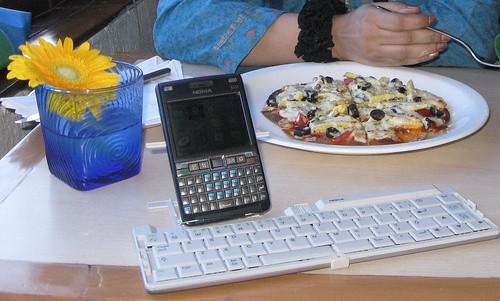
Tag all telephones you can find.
[153,71,273,231]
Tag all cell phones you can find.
[155,73,270,226]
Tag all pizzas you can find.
[261,72,451,147]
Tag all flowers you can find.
[5,37,124,123]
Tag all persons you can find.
[153,0,500,74]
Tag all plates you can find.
[241,61,491,155]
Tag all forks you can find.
[376,4,496,68]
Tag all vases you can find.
[35,62,145,192]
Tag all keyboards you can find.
[133,184,496,296]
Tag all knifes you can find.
[14,68,171,125]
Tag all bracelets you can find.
[294,0,348,61]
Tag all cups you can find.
[35,61,145,190]
